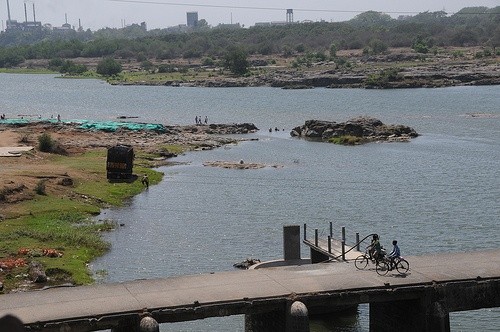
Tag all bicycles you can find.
[354,248,388,270]
[376,255,410,276]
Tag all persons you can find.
[366,234,400,271]
[195,116,202,124]
[0,114,61,122]
[204,116,208,124]
[142,174,148,189]
